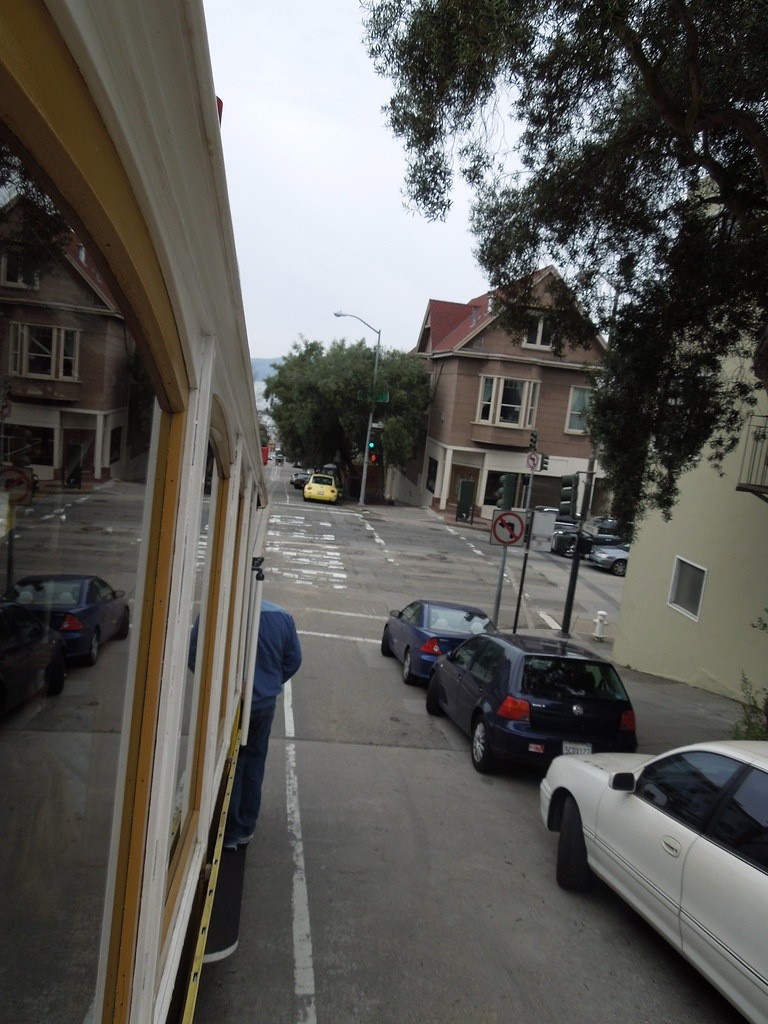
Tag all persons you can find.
[223,598,302,849]
[187,613,199,671]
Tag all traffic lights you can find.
[529,432,538,449]
[559,474,578,517]
[369,439,374,451]
[370,453,381,464]
[13,427,32,466]
[497,474,515,510]
[541,455,549,470]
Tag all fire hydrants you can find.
[592,611,609,643]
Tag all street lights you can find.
[333,312,381,505]
[562,267,621,632]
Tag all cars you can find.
[303,474,338,505]
[290,473,312,490]
[540,740,768,1024]
[551,516,634,558]
[0,600,69,718]
[426,634,639,775]
[381,599,501,686]
[589,544,631,576]
[0,574,130,666]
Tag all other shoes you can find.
[238,833,254,844]
[223,841,237,848]
